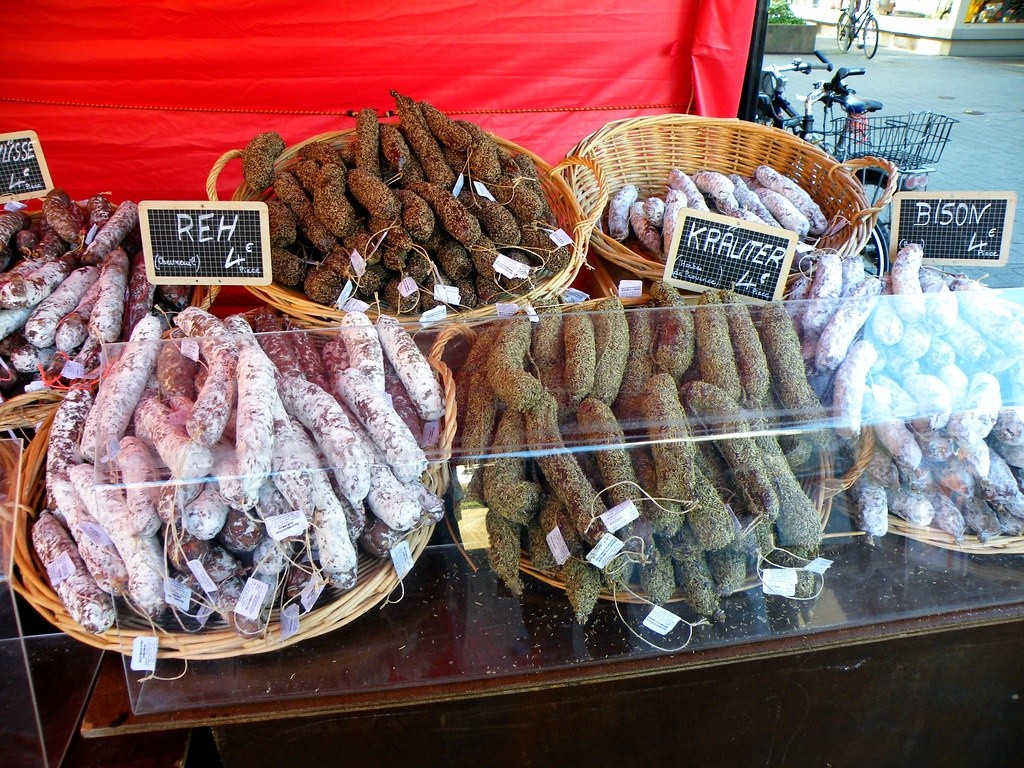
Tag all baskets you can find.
[830,109,962,169]
[0,301,458,662]
[424,290,878,606]
[828,398,1024,556]
[204,124,610,354]
[559,111,898,297]
[1,200,222,432]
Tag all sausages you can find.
[0,87,1022,642]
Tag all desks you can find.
[80,483,1024,736]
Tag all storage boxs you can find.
[561,112,897,305]
[4,307,465,662]
[801,356,1024,557]
[426,288,879,607]
[0,194,224,431]
[205,120,610,348]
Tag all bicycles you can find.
[754,49,960,278]
[834,0,881,58]
